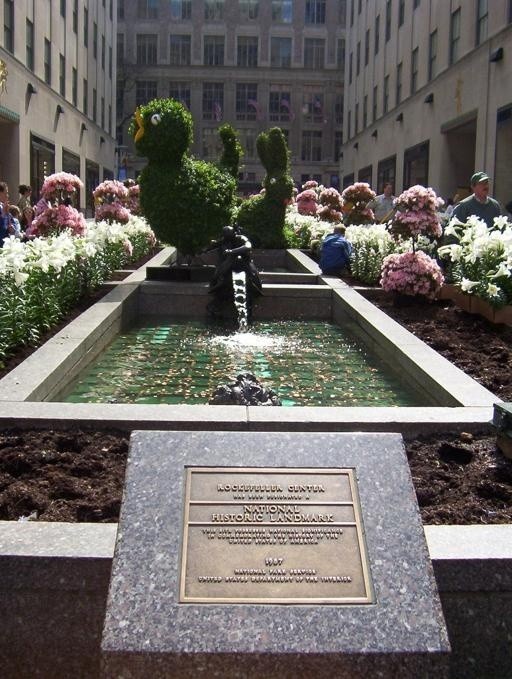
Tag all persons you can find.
[203,226,264,297]
[365,181,400,225]
[1,181,37,249]
[317,222,357,277]
[443,197,454,216]
[448,170,503,235]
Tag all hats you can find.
[471,171,490,185]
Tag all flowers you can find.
[293,180,512,310]
[0,171,139,287]
